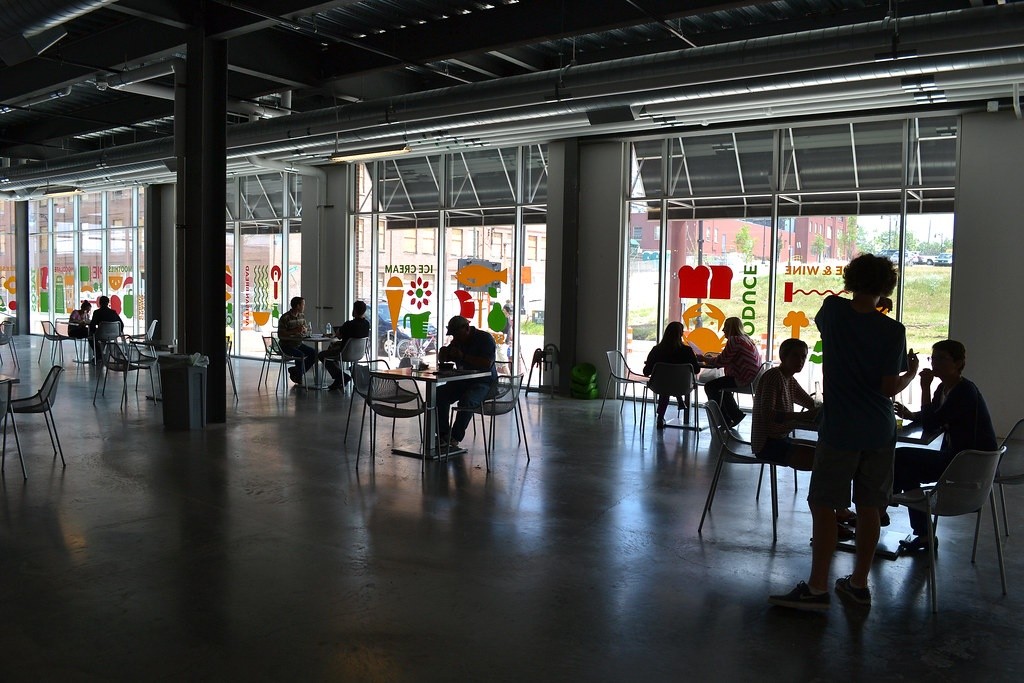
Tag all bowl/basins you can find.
[437,363,454,371]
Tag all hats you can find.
[446,316,471,335]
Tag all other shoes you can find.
[730,410,746,427]
[848,512,891,527]
[288,367,303,384]
[677,403,688,410]
[718,422,734,431]
[327,381,342,390]
[656,418,667,429]
[432,433,459,447]
[837,511,857,540]
[899,537,939,552]
[339,373,351,390]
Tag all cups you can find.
[168,345,177,354]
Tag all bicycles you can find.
[397,330,437,361]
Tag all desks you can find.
[663,363,722,430]
[298,334,344,392]
[370,369,493,460]
[62,319,95,364]
[119,340,179,411]
[0,375,28,480]
[793,418,943,559]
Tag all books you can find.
[690,338,703,356]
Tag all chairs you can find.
[0,318,239,468]
[259,332,532,475]
[607,332,1023,613]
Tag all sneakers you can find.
[768,580,831,609]
[835,574,871,604]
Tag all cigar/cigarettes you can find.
[912,352,920,355]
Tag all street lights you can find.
[934,233,943,253]
[881,215,891,248]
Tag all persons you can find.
[68,300,90,340]
[769,254,918,608]
[697,317,763,431]
[501,304,514,375]
[278,297,316,383]
[435,316,499,447]
[90,296,124,365]
[318,300,370,389]
[643,321,701,426]
[850,340,999,552]
[749,339,856,542]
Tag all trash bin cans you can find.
[5,317,17,335]
[155,354,206,431]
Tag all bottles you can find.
[814,381,824,408]
[326,322,332,334]
[306,322,312,337]
[84,310,87,321]
[891,392,904,426]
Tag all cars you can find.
[874,249,939,266]
[934,253,953,265]
[360,299,437,356]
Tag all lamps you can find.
[43,187,84,199]
[586,104,647,126]
[331,140,412,163]
